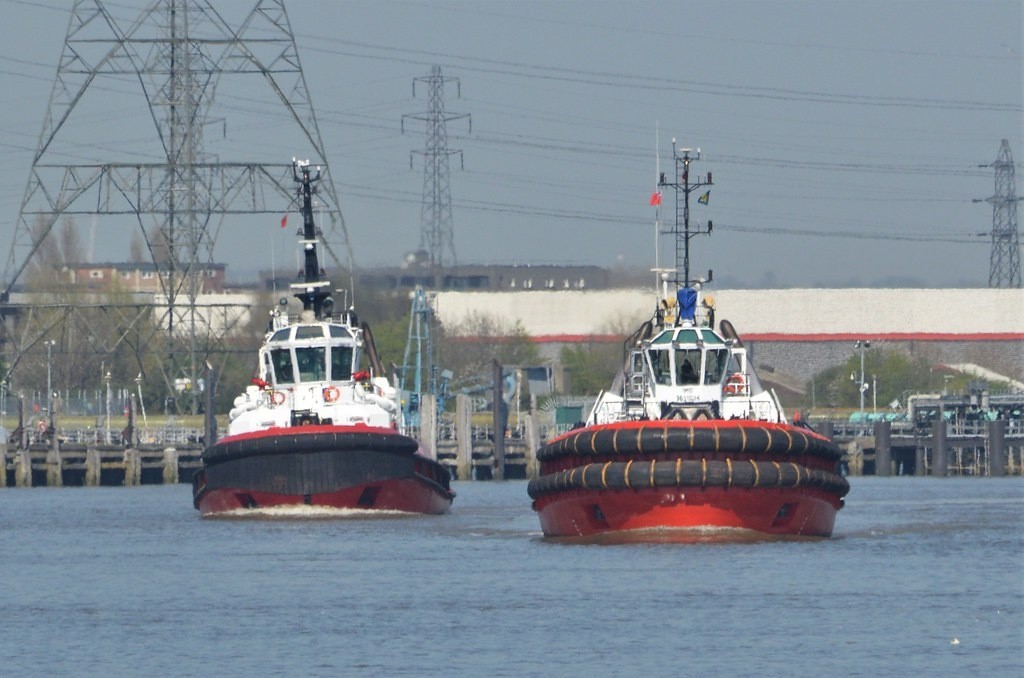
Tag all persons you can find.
[681,359,693,373]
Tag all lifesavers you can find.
[727,375,745,392]
[271,391,286,405]
[323,384,341,403]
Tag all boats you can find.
[528,118,850,546]
[192,155,458,518]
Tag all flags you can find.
[281,213,287,228]
[698,190,711,205]
[650,192,661,206]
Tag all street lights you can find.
[850,339,870,433]
[44,340,56,427]
[104,371,113,445]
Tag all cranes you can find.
[395,286,516,429]
